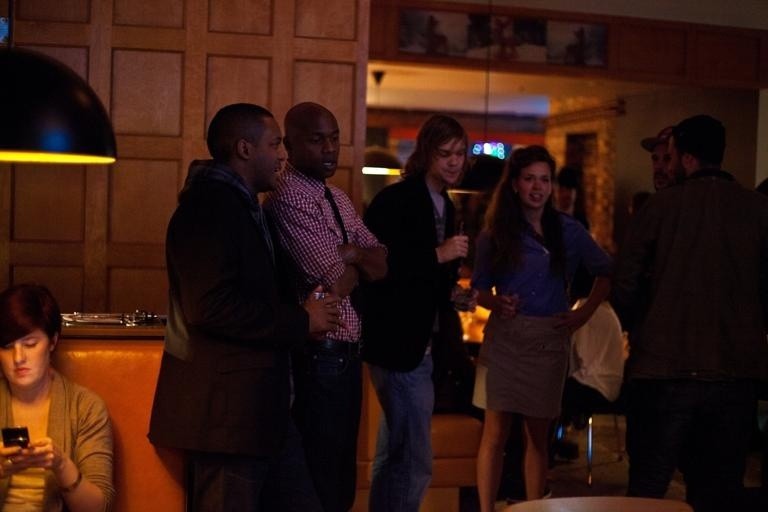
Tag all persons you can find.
[348,113,480,512]
[261,102,389,511]
[505,273,626,504]
[608,112,768,512]
[554,165,591,231]
[471,143,615,512]
[0,281,117,512]
[147,100,348,512]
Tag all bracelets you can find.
[55,472,82,494]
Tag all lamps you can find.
[0,43,118,166]
[461,1,506,182]
[360,70,403,176]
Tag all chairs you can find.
[356,362,501,512]
[587,331,632,487]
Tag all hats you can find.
[640,125,672,150]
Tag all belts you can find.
[312,339,364,354]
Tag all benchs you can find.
[52,339,189,512]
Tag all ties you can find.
[324,186,348,244]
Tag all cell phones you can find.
[1,425,31,447]
